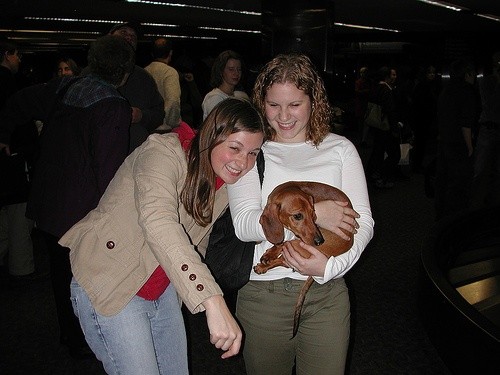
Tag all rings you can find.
[293,267,297,272]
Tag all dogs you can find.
[253,180,354,277]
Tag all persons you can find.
[58,97,266,375]
[201,50,251,311]
[1,18,191,279]
[225,55,375,374]
[25,32,136,361]
[354,55,500,215]
